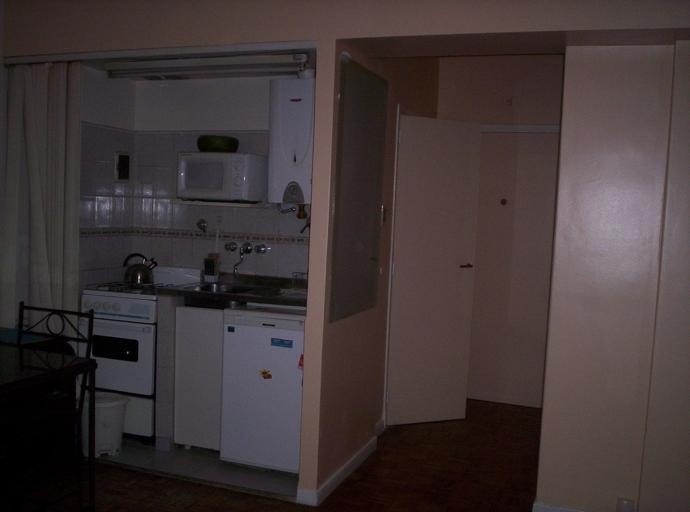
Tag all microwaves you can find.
[177,152,267,203]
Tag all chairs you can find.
[17,299,95,510]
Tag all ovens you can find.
[77,317,155,439]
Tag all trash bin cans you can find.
[76,392,129,458]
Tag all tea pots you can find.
[122,253,159,285]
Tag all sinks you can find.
[196,281,252,294]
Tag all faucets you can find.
[231,247,245,279]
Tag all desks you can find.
[0,342,98,509]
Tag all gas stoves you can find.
[80,279,177,323]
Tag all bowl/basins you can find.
[197,135,238,153]
